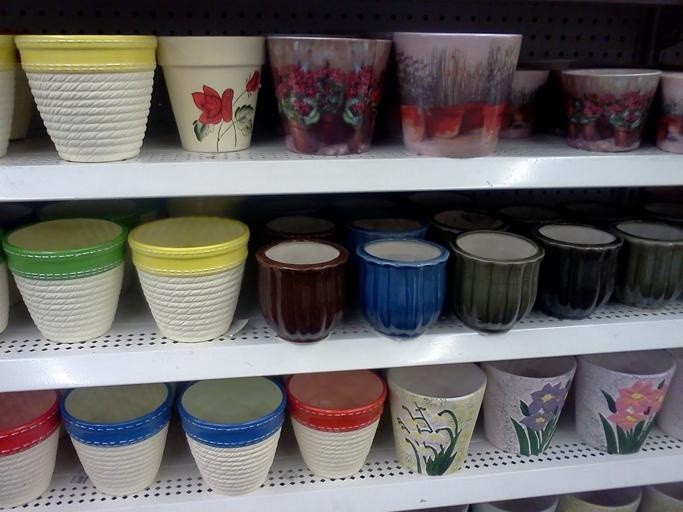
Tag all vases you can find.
[428,482,679,511]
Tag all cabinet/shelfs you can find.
[0,0,681,511]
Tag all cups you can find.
[1,351,683,512]
[2,33,683,155]
[0,217,682,346]
